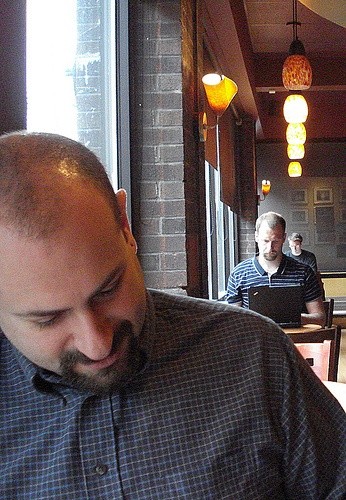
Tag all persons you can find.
[284,233,317,275]
[1,132,346,500]
[227,211,326,328]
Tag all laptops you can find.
[248,285,302,328]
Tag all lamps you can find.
[283,39,313,177]
[198,74,239,142]
[258,180,271,206]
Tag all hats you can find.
[288,233,302,241]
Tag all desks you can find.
[282,326,341,382]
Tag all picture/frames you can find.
[289,187,346,258]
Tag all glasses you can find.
[290,245,299,248]
